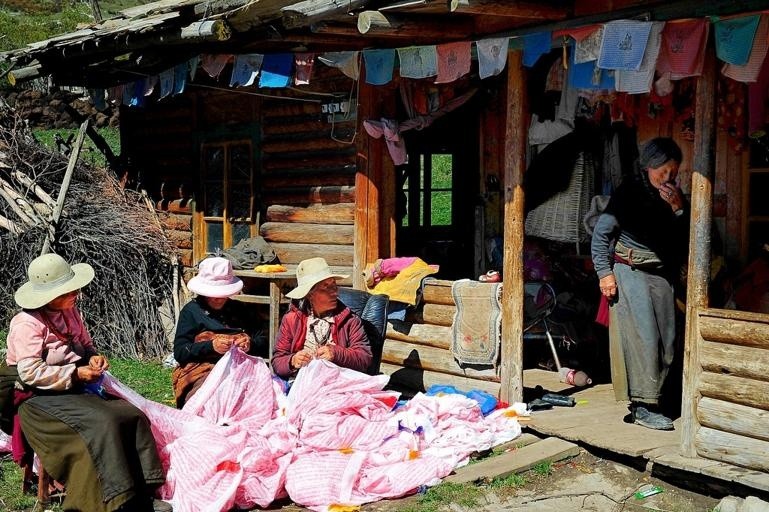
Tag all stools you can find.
[21,444,69,511]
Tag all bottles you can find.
[557,367,592,386]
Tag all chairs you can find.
[336,286,390,376]
[484,236,603,377]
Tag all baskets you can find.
[524,140,598,244]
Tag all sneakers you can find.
[479,270,501,284]
[631,402,676,431]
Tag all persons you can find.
[7,253,172,511]
[272,257,374,396]
[172,257,269,410]
[591,137,689,429]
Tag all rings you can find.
[244,344,249,348]
[666,196,669,198]
[669,191,672,195]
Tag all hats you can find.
[187,257,244,299]
[285,255,350,300]
[14,253,97,310]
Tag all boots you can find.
[522,384,577,412]
